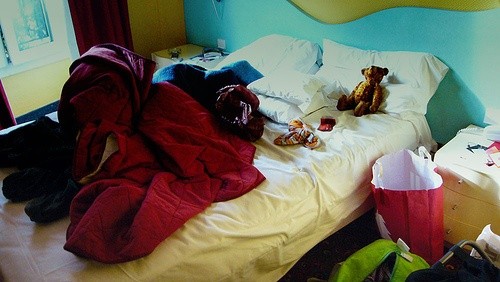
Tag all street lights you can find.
[306,238,431,282]
[370,146,445,265]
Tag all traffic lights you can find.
[247,71,323,125]
[316,38,450,114]
[214,33,321,75]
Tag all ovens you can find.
[0,65,438,282]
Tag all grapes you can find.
[149,43,203,71]
[434,122,499,261]
